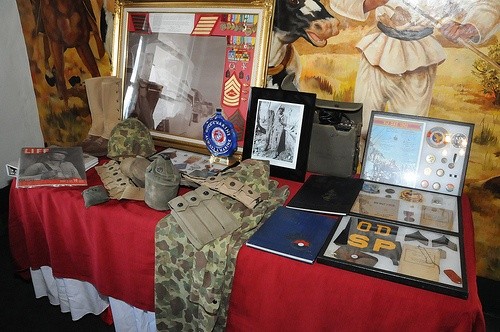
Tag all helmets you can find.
[107,117,157,157]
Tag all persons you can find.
[22,148,83,180]
[270,107,287,159]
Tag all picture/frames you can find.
[242,87,316,183]
[110,0,276,161]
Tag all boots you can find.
[127,79,164,130]
[72,77,122,152]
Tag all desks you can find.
[7,157,486,332]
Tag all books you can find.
[16,147,87,187]
[246,206,338,265]
[286,174,365,216]
